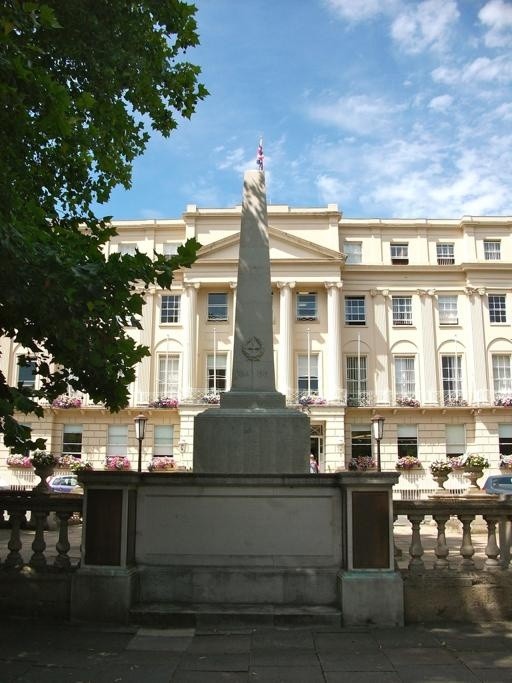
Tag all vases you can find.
[31,464,54,491]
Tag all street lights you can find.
[133,411,149,473]
[370,410,386,472]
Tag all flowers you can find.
[427,453,487,493]
[30,450,57,463]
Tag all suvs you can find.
[47,474,84,521]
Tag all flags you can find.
[256,143,263,170]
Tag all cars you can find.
[482,474,511,523]
